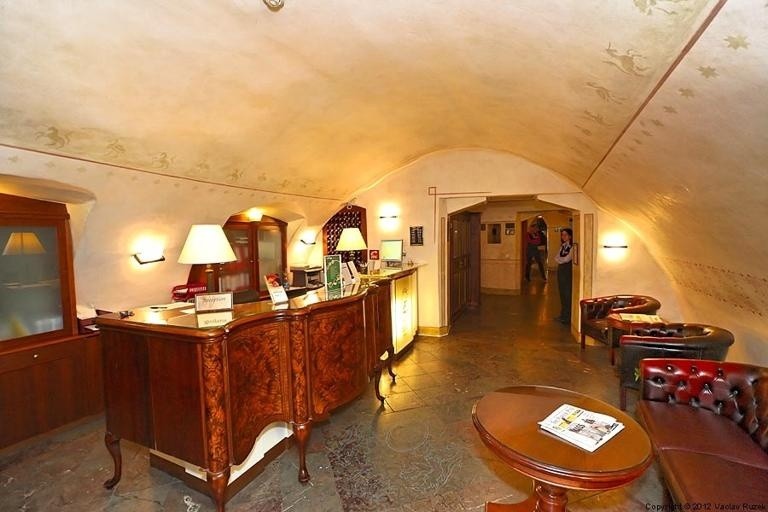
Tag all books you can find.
[535,402,626,452]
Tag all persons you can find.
[526,222,547,281]
[553,229,573,323]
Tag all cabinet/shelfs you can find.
[257,222,285,300]
[215,219,257,291]
[79,336,105,417]
[0,191,79,449]
[449,211,483,325]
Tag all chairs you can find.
[616,319,734,412]
[579,293,661,367]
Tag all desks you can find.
[469,384,655,512]
[290,267,324,287]
[604,312,665,366]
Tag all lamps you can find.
[178,223,238,294]
[2,231,46,257]
[335,227,368,285]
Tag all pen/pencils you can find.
[150,306,168,309]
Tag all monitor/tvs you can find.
[380,240,403,262]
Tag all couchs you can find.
[635,357,768,511]
